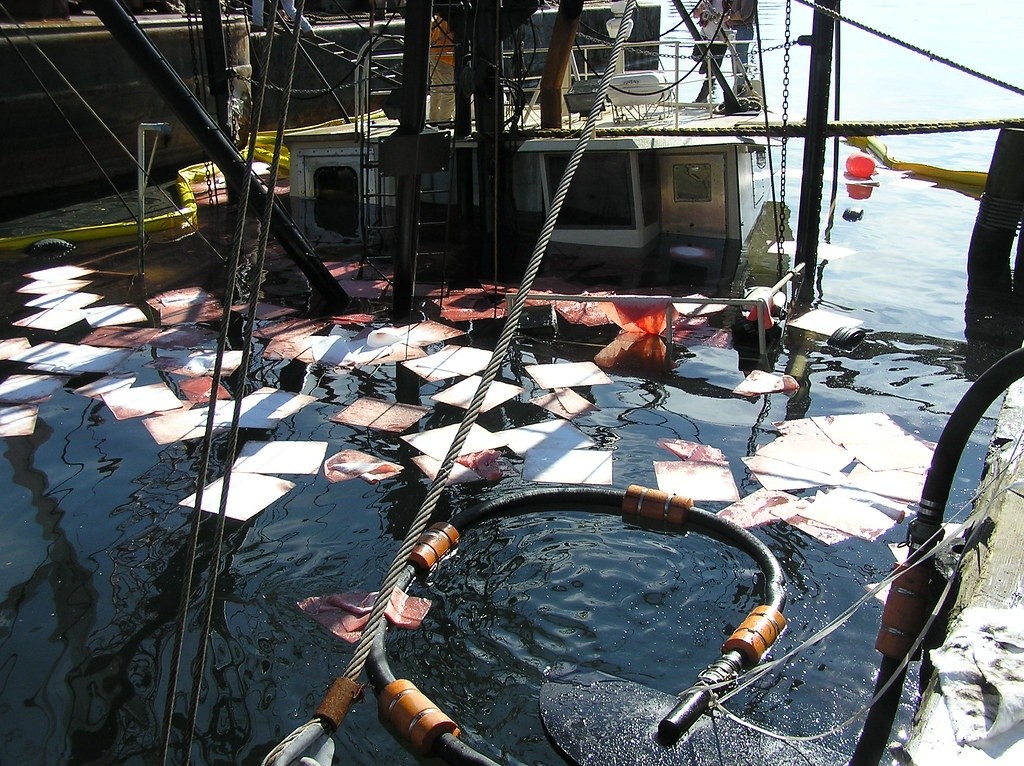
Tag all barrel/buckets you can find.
[847,153,875,178]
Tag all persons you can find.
[251,0,315,39]
[724,0,758,97]
[429,17,456,130]
[692,0,728,103]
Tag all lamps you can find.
[380,89,401,122]
[563,79,606,118]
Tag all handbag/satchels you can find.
[691,35,709,62]
